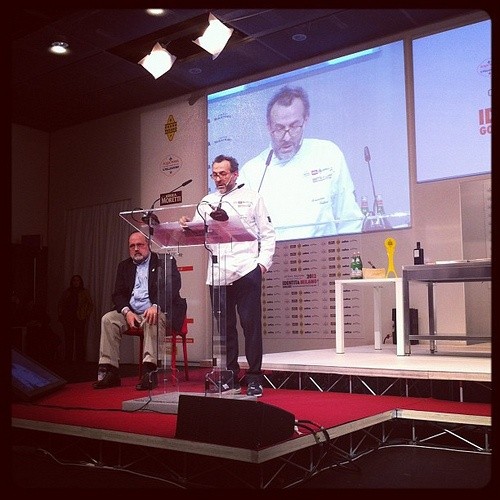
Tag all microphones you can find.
[141,180,192,225]
[210,183,245,221]
[364,145,378,217]
[258,149,273,192]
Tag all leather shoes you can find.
[93,372,120,389]
[135,368,157,389]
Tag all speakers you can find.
[175,395,295,450]
[8,356,68,398]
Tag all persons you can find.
[178,156,276,397]
[235,86,364,241]
[93,231,187,391]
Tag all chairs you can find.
[123,298,189,386]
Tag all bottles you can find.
[356,252,363,279]
[413,241,424,265]
[350,252,356,279]
[373,194,389,230]
[359,197,372,232]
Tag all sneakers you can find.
[211,382,240,396]
[247,382,263,397]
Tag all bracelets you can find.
[123,308,130,316]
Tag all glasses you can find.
[210,171,231,180]
[267,120,305,139]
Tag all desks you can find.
[401,262,492,357]
[335,277,405,356]
